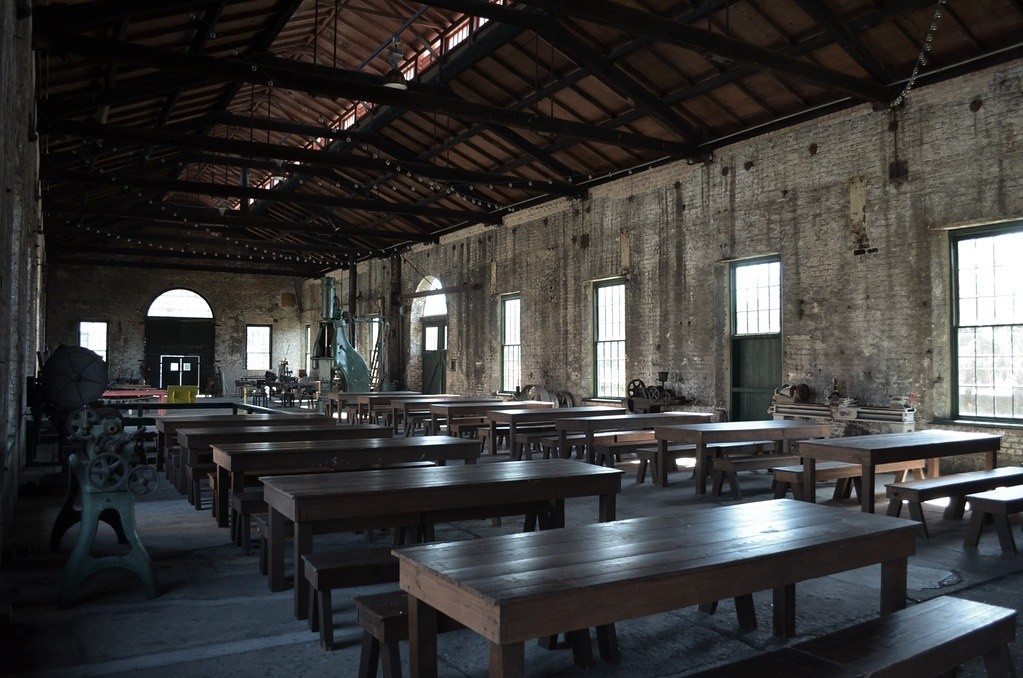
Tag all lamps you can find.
[378,30,411,94]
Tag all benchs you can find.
[682,595,1019,678]
[354,588,758,678]
[633,438,778,486]
[302,543,399,651]
[963,485,1023,555]
[770,457,927,505]
[711,452,843,501]
[885,465,1023,539]
[254,512,435,576]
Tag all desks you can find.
[391,497,924,678]
[258,458,627,621]
[770,401,916,474]
[96,370,713,540]
[654,419,834,498]
[796,429,1001,514]
